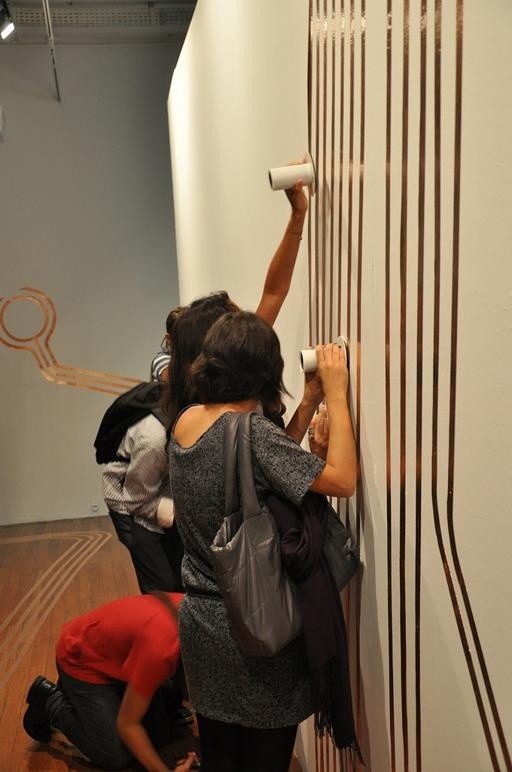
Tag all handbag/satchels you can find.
[208,407,363,660]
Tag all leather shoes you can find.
[22,673,57,745]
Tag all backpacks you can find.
[91,378,176,466]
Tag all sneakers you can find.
[173,703,195,726]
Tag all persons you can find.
[103,400,180,594]
[151,307,191,382]
[26,592,201,772]
[170,313,357,772]
[162,162,324,455]
